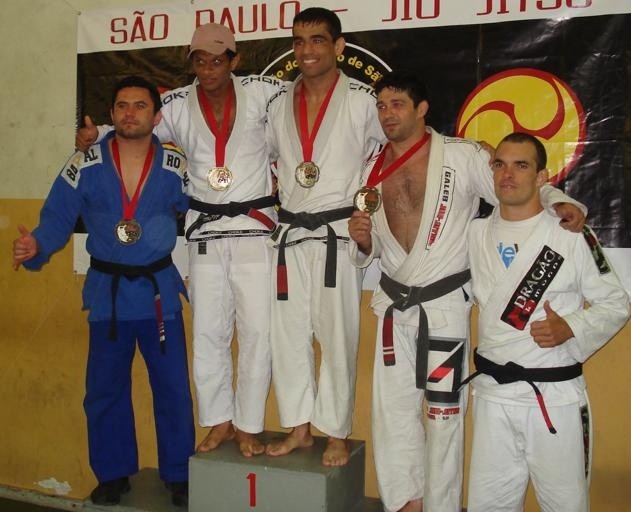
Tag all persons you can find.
[12,74,277,508]
[266,6,496,467]
[347,72,589,512]
[74,23,295,457]
[467,131,631,511]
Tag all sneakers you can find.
[172,482,188,508]
[90,472,130,506]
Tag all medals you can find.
[206,166,233,192]
[353,185,382,215]
[295,161,319,188]
[113,219,143,246]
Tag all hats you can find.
[187,22,237,59]
